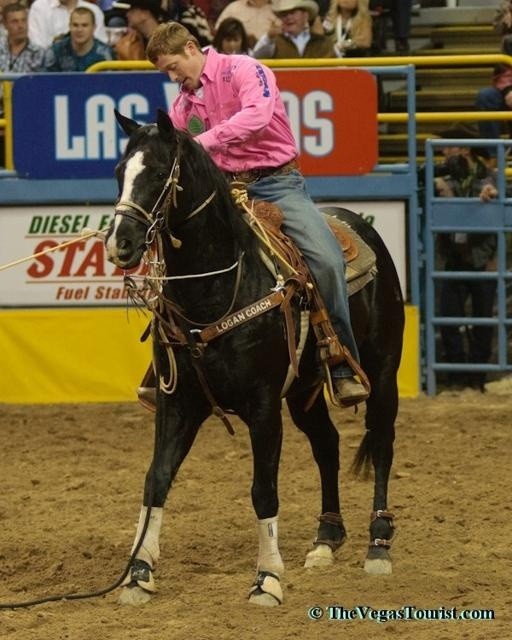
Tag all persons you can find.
[136,21,368,407]
[1,0,420,175]
[416,121,508,392]
[477,176,512,202]
[473,32,512,169]
[493,0,512,36]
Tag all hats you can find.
[273,0,319,20]
[432,121,489,159]
[117,0,167,16]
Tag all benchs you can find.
[376,25,512,180]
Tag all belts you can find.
[230,159,298,186]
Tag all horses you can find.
[101,105,407,610]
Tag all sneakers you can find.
[136,386,158,405]
[334,377,368,403]
